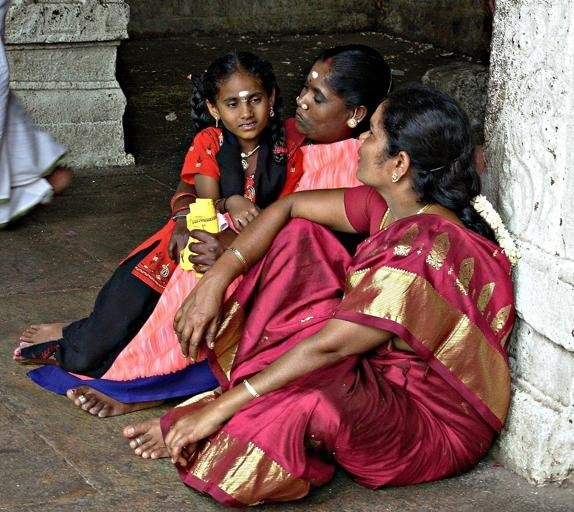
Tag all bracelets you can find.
[167,189,228,221]
[226,241,250,273]
[243,380,261,400]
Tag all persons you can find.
[0,40,72,227]
[123,83,516,508]
[12,50,286,375]
[66,43,394,417]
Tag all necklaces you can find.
[231,142,262,171]
[378,199,431,232]
